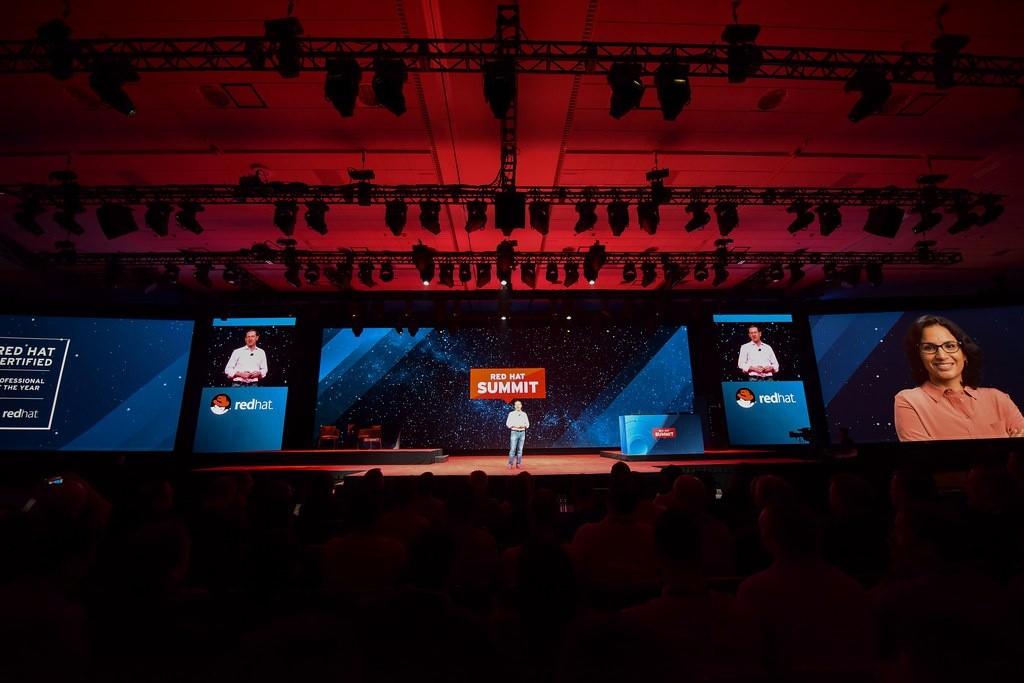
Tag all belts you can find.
[749,376,773,380]
[511,430,524,432]
[232,381,256,386]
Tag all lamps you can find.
[12,15,1009,293]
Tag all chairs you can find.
[355,428,382,449]
[318,425,339,449]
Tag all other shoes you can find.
[508,464,513,469]
[516,464,520,468]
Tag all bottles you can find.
[557,494,568,512]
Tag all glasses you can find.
[916,341,962,354]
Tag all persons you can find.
[738,327,779,382]
[894,316,1024,441]
[0,428,1024,682]
[225,330,268,388]
[506,402,529,468]
